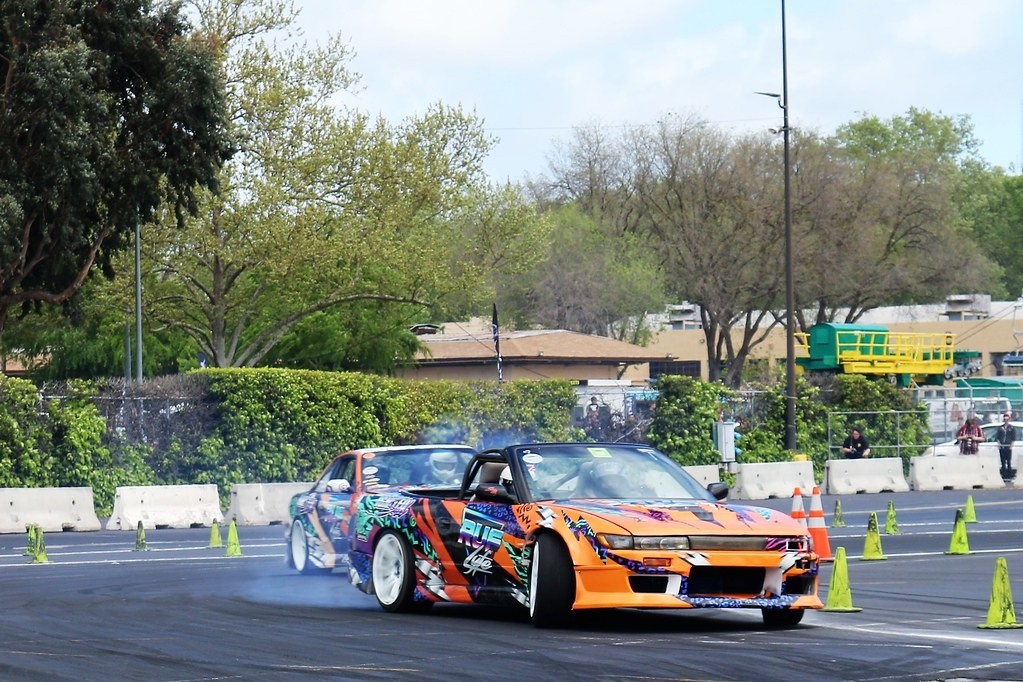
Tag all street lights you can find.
[755,1,800,451]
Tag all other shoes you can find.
[1002,477,1012,482]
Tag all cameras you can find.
[966,438,973,449]
[850,448,857,454]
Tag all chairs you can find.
[481,462,507,484]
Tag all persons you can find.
[842,427,870,459]
[569,457,643,498]
[499,465,544,499]
[997,414,1016,472]
[421,450,460,484]
[956,418,985,455]
[574,397,657,444]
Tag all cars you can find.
[923,419,1022,458]
[287,445,482,574]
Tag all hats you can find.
[588,458,629,483]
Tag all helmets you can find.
[429,452,458,481]
[499,465,538,484]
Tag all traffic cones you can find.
[962,495,978,525]
[814,548,863,612]
[223,520,245,556]
[802,487,836,561]
[25,527,53,563]
[206,518,227,548]
[945,508,973,556]
[976,557,1023,629]
[857,515,889,561]
[883,499,901,534]
[131,521,151,553]
[22,524,38,556]
[830,499,845,528]
[352,443,825,630]
[791,487,808,530]
